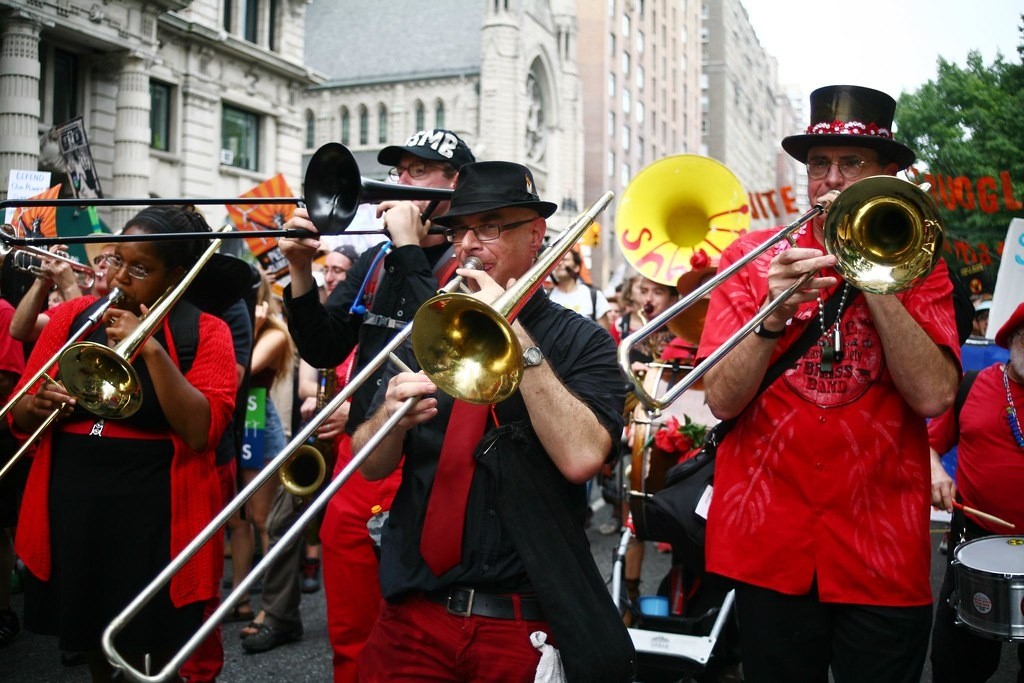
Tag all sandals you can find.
[222,598,255,621]
[240,609,269,640]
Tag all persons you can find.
[695,84,963,683]
[971,293,993,336]
[179,244,361,683]
[352,159,640,683]
[925,302,1024,683]
[0,223,127,648]
[5,202,238,683]
[545,246,720,634]
[276,127,477,683]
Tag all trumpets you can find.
[0,222,105,287]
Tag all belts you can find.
[422,579,548,621]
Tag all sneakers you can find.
[241,625,303,652]
[0,611,22,647]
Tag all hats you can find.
[994,301,1024,349]
[431,161,558,226]
[781,84,917,170]
[378,128,476,171]
[970,292,993,311]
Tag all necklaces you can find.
[1003,359,1024,448]
[816,272,850,372]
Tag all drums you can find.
[949,534,1024,639]
[625,358,734,542]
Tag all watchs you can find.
[753,312,786,339]
[519,346,544,368]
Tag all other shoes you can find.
[299,556,320,593]
[599,517,621,536]
[223,542,232,557]
[63,651,90,667]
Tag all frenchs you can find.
[614,153,752,356]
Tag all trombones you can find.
[0,142,455,246]
[615,176,945,410]
[0,223,230,473]
[101,190,612,683]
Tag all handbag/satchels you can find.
[627,444,727,584]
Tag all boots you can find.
[249,553,265,593]
[620,577,641,628]
[222,576,235,589]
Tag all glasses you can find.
[806,153,884,179]
[445,218,535,241]
[388,160,432,182]
[1015,328,1024,345]
[94,254,108,264]
[104,243,170,280]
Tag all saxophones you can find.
[278,357,342,547]
